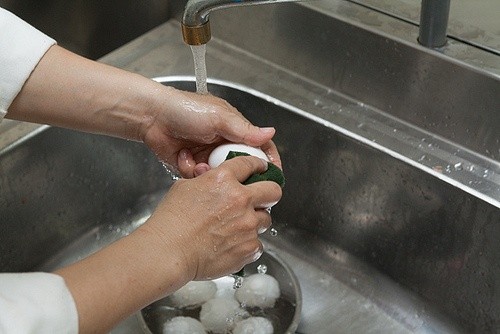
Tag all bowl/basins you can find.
[136,249,303,334]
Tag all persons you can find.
[0,5,286,334]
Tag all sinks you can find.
[0,22,499,334]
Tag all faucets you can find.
[180,0,453,49]
